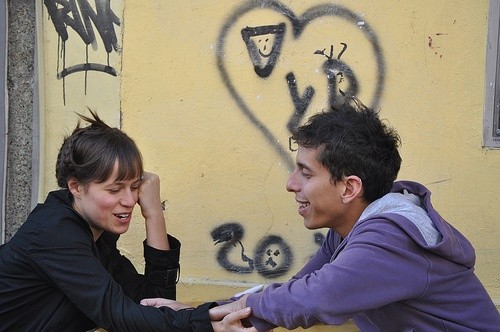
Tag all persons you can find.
[137,101,500,332]
[0,106,259,332]
